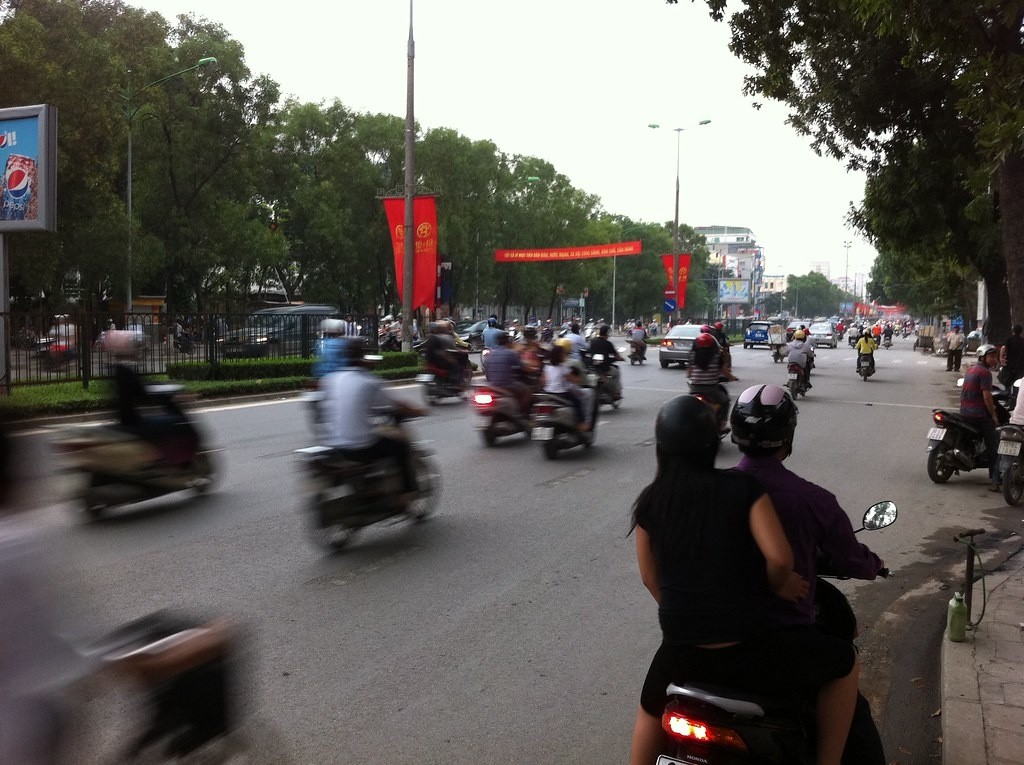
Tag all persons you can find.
[853,329,877,373]
[624,318,692,338]
[959,325,1024,491]
[1,406,244,764]
[837,322,844,336]
[946,326,963,372]
[626,320,647,359]
[428,314,623,439]
[848,322,893,347]
[688,321,740,436]
[314,319,430,517]
[784,325,816,387]
[345,311,418,358]
[895,319,915,333]
[630,384,886,765]
[109,340,211,488]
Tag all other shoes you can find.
[580,423,592,430]
[807,381,812,388]
[643,357,647,360]
[987,483,1000,491]
[721,427,730,436]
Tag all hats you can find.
[863,329,869,334]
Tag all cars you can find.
[742,320,770,348]
[808,322,840,348]
[785,321,812,343]
[659,324,705,369]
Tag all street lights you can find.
[648,119,712,323]
[124,57,218,312]
[843,240,853,319]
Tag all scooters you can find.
[785,346,816,401]
[44,381,221,526]
[351,323,666,462]
[853,344,879,382]
[287,371,445,553]
[769,313,920,350]
[923,377,1021,486]
[31,330,149,371]
[687,375,738,440]
[162,328,196,356]
[654,500,900,765]
[993,422,1024,507]
[44,598,294,765]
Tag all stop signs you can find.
[663,285,676,299]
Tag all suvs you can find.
[215,303,348,360]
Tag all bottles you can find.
[948,596,968,642]
[948,592,960,621]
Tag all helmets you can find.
[493,322,572,352]
[701,324,712,333]
[696,334,714,348]
[636,320,642,326]
[730,383,797,452]
[796,325,802,330]
[326,336,362,363]
[106,330,144,357]
[867,328,871,332]
[655,394,720,452]
[487,318,496,326]
[800,324,806,329]
[976,344,994,360]
[323,319,346,335]
[431,320,453,334]
[795,330,805,338]
[714,322,723,330]
[803,328,809,335]
